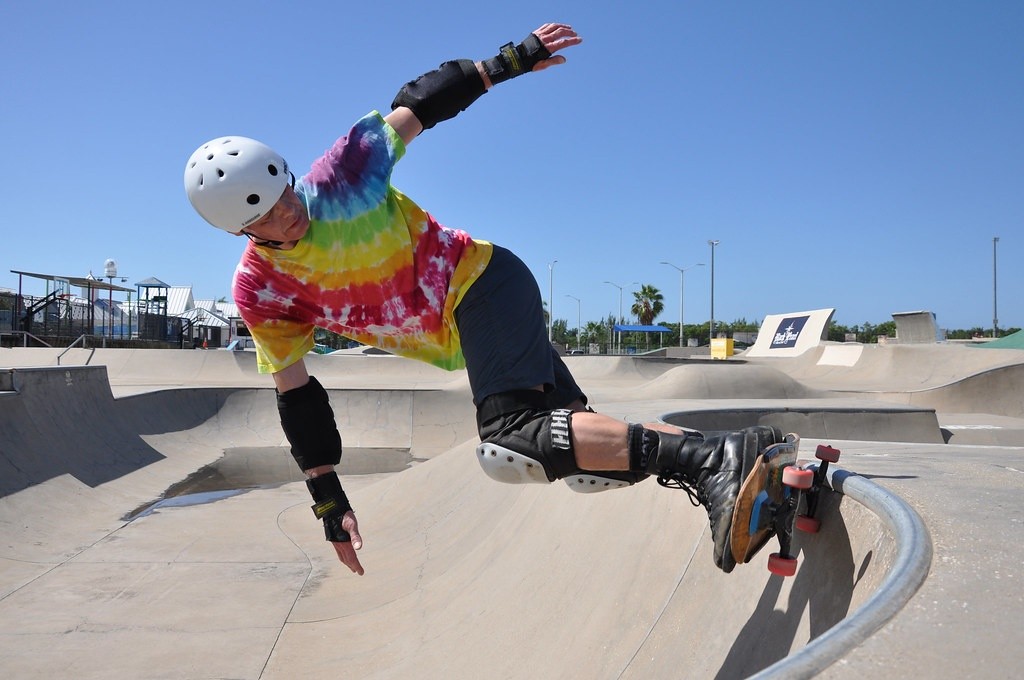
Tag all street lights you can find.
[565,295,580,351]
[661,262,706,348]
[604,282,638,355]
[549,260,558,345]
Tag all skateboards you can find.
[726,426,844,581]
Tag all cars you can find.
[570,350,584,356]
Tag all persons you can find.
[181,21,785,574]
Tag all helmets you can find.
[184,136,289,234]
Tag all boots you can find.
[645,424,783,573]
